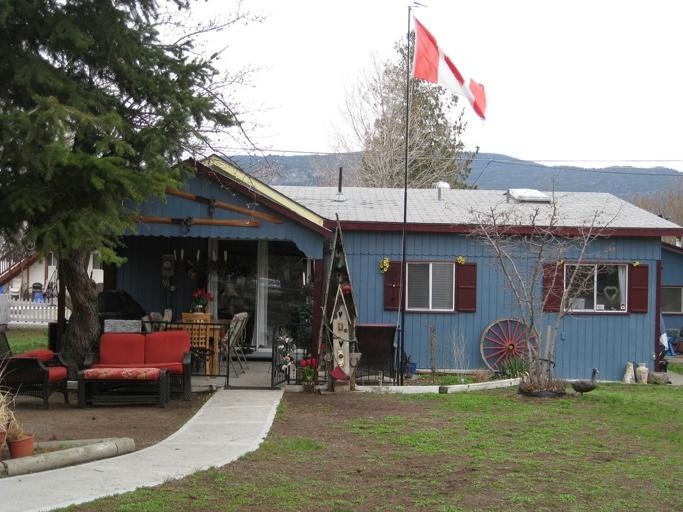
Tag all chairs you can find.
[1,331,70,409]
[83,330,191,408]
[141,309,250,379]
[8,278,22,302]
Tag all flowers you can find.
[189,287,214,304]
[300,357,318,384]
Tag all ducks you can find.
[571,367,600,397]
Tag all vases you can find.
[194,304,205,313]
[6,431,34,458]
[303,384,315,393]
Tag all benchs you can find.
[76,368,171,408]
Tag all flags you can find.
[410,12,486,119]
